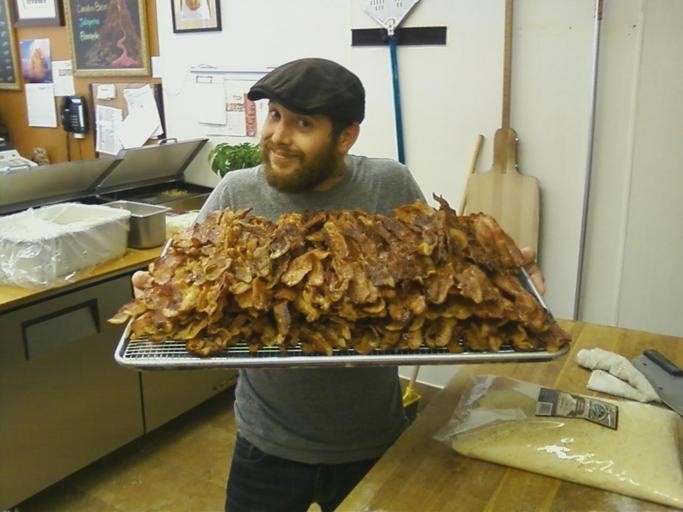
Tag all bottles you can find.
[248,58,365,123]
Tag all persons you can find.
[131,57,546,512]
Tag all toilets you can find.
[59,96,89,133]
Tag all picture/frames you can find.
[330,319,681,512]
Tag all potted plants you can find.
[0,1,222,92]
